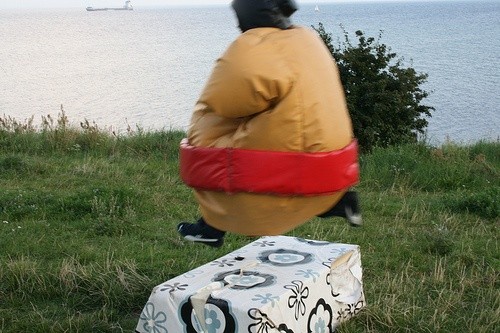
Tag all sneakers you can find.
[339,192,364,227]
[177,222,224,248]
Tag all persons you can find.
[176,0,363,249]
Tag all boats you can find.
[86,1,134,11]
